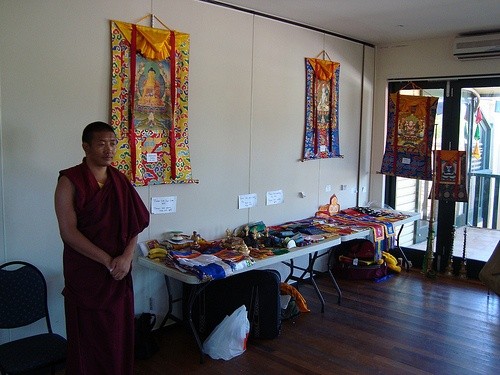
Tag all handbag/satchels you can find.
[202,305,249,361]
[227,270,281,338]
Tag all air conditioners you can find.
[452,33,500,60]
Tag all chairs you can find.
[0,260,69,375]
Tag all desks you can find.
[308,207,422,306]
[137,220,342,366]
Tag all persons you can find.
[53,121,151,375]
